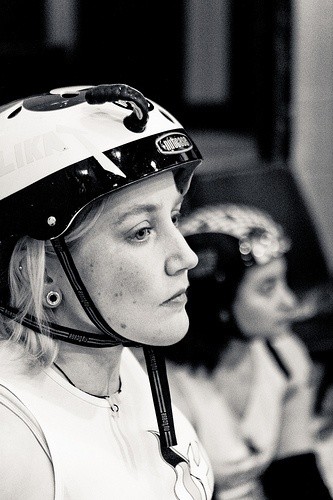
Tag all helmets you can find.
[167,204,289,332]
[0,82,202,308]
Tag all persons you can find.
[128,203,333,500]
[0,81,219,500]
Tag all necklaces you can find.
[50,360,122,414]
